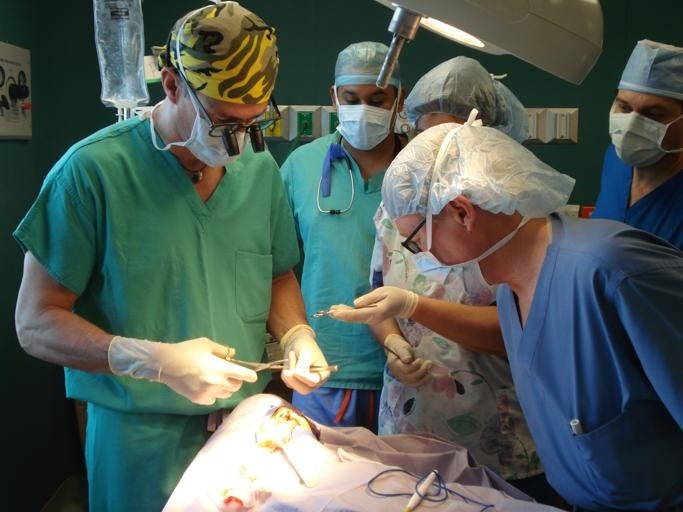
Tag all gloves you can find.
[330,286,420,325]
[382,333,433,391]
[278,323,332,398]
[107,334,259,406]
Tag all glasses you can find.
[401,217,429,256]
[179,72,283,137]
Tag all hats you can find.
[619,38,683,102]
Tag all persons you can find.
[377,122,683,510]
[326,55,569,486]
[5,3,333,512]
[587,37,683,248]
[277,38,423,428]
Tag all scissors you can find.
[218,343,289,373]
[312,304,378,317]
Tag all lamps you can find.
[373,0,605,89]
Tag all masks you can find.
[336,103,392,150]
[183,109,247,168]
[607,114,667,166]
[410,251,493,306]
